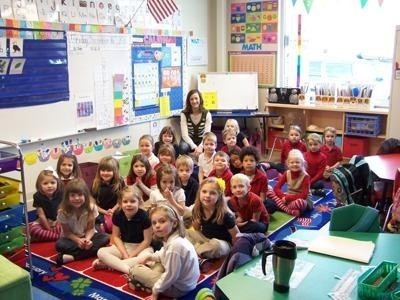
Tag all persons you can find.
[129,201,200,300]
[139,134,160,170]
[90,156,125,225]
[153,126,180,159]
[150,164,185,222]
[29,170,65,242]
[276,126,307,174]
[185,177,241,273]
[56,153,82,187]
[179,89,212,154]
[154,143,176,170]
[301,133,327,197]
[208,151,233,196]
[198,132,217,186]
[229,146,244,173]
[92,185,154,274]
[224,119,249,147]
[220,128,242,153]
[266,149,313,216]
[319,127,343,172]
[124,154,157,201]
[225,147,276,215]
[175,155,198,206]
[223,173,269,234]
[55,178,110,266]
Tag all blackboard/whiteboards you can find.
[196,71,258,111]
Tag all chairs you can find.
[377,138,399,154]
[349,154,388,200]
[331,204,381,232]
[199,287,212,299]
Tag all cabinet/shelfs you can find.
[264,102,390,159]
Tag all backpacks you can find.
[330,155,372,206]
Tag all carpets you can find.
[25,139,337,299]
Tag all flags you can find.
[147,0,180,24]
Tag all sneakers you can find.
[316,188,326,198]
[199,259,215,273]
[127,280,152,294]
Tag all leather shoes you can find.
[56,254,65,267]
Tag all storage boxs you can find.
[346,115,383,137]
[268,129,282,148]
[343,138,370,157]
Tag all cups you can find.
[262,239,297,294]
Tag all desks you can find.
[359,153,400,180]
[175,109,279,153]
[216,229,400,300]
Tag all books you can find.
[307,233,375,264]
